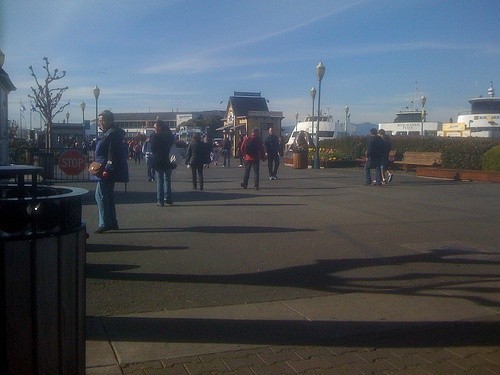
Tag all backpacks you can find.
[245,135,263,160]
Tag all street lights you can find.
[80,100,86,126]
[93,84,100,142]
[313,61,325,169]
[421,96,426,136]
[311,86,316,148]
[345,105,349,132]
[66,111,70,125]
[295,113,299,135]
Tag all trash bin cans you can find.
[0,166,90,375]
[33,152,54,180]
[293,148,308,169]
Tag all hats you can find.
[99,110,114,120]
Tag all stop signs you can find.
[59,150,86,175]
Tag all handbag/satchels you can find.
[168,162,177,169]
[88,162,103,176]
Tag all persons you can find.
[237,136,245,168]
[135,142,142,164]
[265,128,284,181]
[152,120,173,208]
[95,110,129,234]
[142,133,156,183]
[363,128,385,186]
[241,127,267,190]
[64,134,96,159]
[379,129,394,185]
[185,133,207,191]
[212,140,222,167]
[221,137,231,168]
[204,138,212,168]
[123,136,135,160]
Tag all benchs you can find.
[356,150,442,176]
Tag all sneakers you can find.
[156,202,164,206]
[383,177,386,181]
[373,180,377,182]
[164,199,173,204]
[388,173,393,182]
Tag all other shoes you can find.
[94,227,110,233]
[241,182,248,189]
[107,223,118,230]
[254,185,258,190]
[374,182,381,186]
[273,176,277,179]
[363,183,371,186]
[269,177,273,180]
